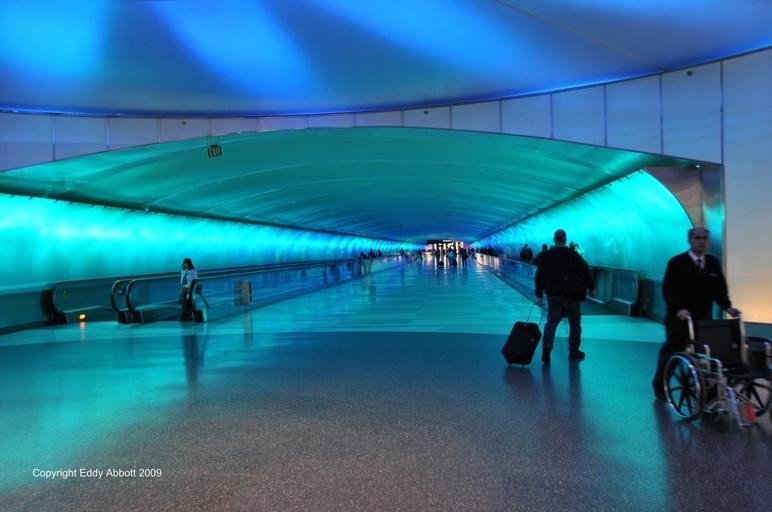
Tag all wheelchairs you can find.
[663,312,771,430]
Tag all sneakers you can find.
[653,378,666,400]
[569,350,585,360]
[542,353,550,363]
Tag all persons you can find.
[535,244,548,265]
[360,245,494,266]
[652,228,740,421]
[180,259,198,320]
[535,229,596,364]
[520,244,533,264]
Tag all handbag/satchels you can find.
[563,271,588,302]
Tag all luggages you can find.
[501,303,545,367]
